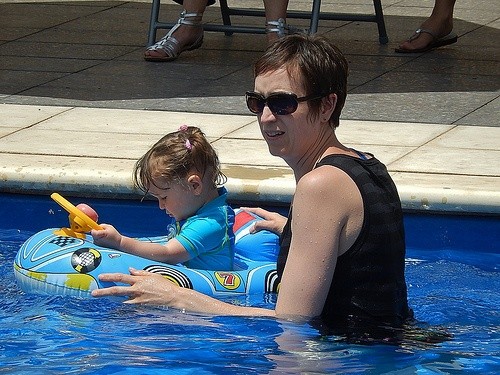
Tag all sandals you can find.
[143,9,204,61]
[265,18,289,38]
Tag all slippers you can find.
[395,28,457,53]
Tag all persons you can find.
[395,0,457,53]
[143,0,289,62]
[90,125,235,272]
[92,32,411,318]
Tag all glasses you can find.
[245,90,330,116]
